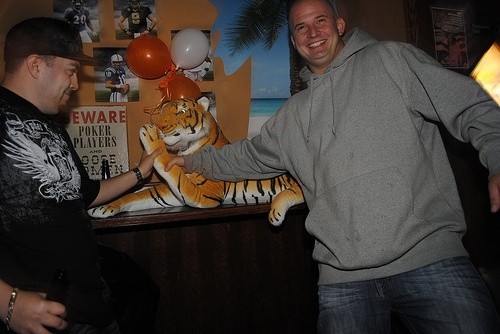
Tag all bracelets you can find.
[5,287,19,333]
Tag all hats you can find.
[2,17,107,66]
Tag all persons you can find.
[104,53,131,105]
[0,16,163,334]
[62,1,97,41]
[163,0,500,334]
[184,56,211,79]
[117,1,158,40]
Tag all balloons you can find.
[168,25,212,72]
[123,32,174,80]
[161,74,201,102]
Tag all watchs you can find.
[131,166,145,186]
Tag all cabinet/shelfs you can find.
[89,203,320,334]
